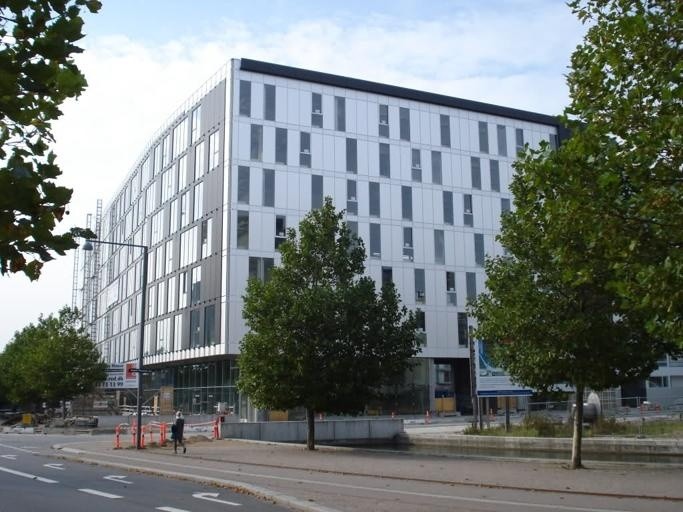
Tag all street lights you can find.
[82,238,148,450]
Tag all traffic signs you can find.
[128,369,152,372]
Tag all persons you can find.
[171,411,186,454]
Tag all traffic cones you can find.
[488,407,495,422]
[424,410,431,424]
